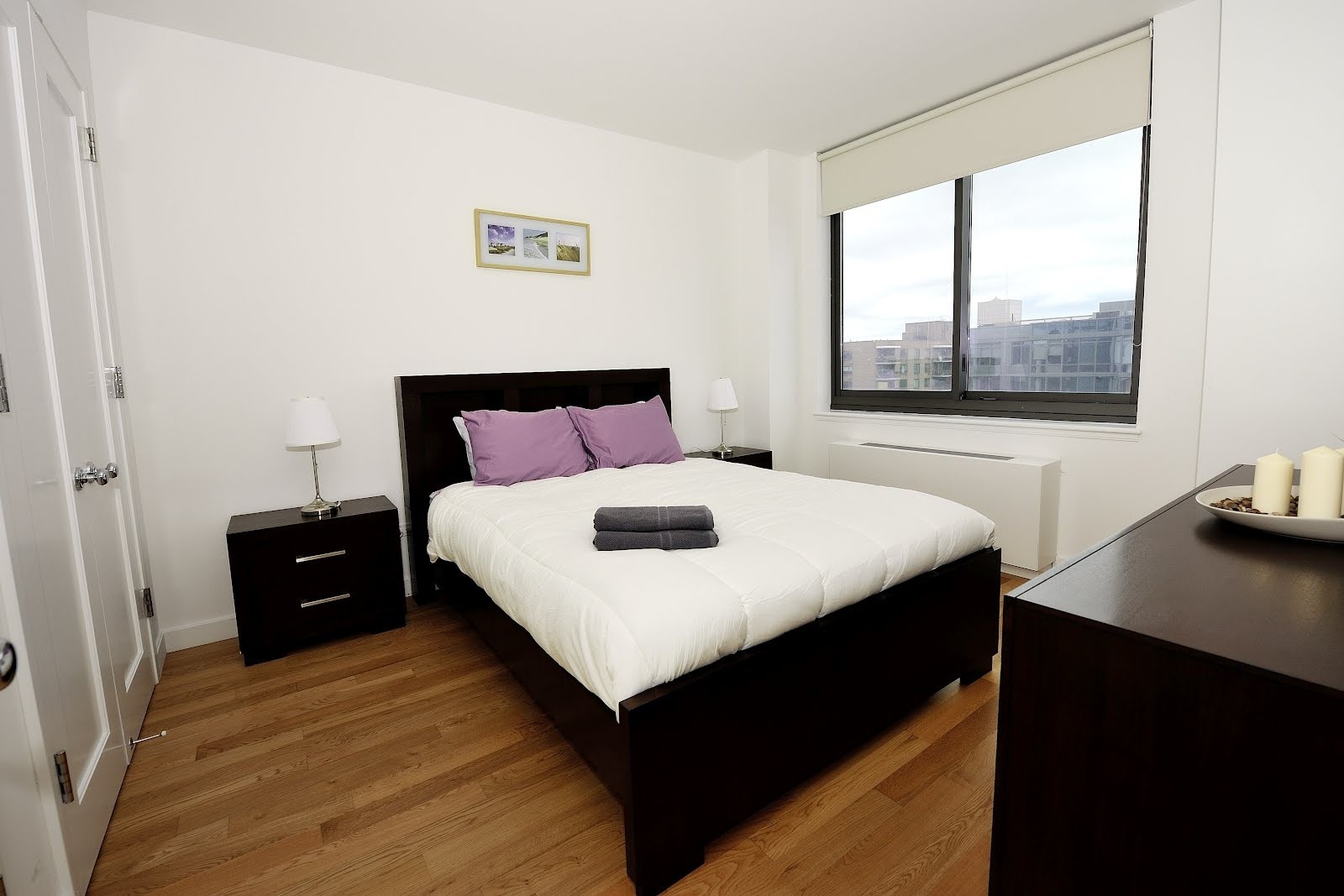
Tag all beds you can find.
[394,368,1003,896]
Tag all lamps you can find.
[286,396,341,516]
[708,378,739,456]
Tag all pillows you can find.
[566,395,685,469]
[453,410,596,487]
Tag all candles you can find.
[1252,449,1294,513]
[1297,446,1344,518]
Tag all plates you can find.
[1196,484,1344,542]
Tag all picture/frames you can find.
[475,208,591,276]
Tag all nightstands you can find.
[684,446,772,469]
[226,496,407,667]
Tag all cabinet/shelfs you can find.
[989,464,1343,896]
